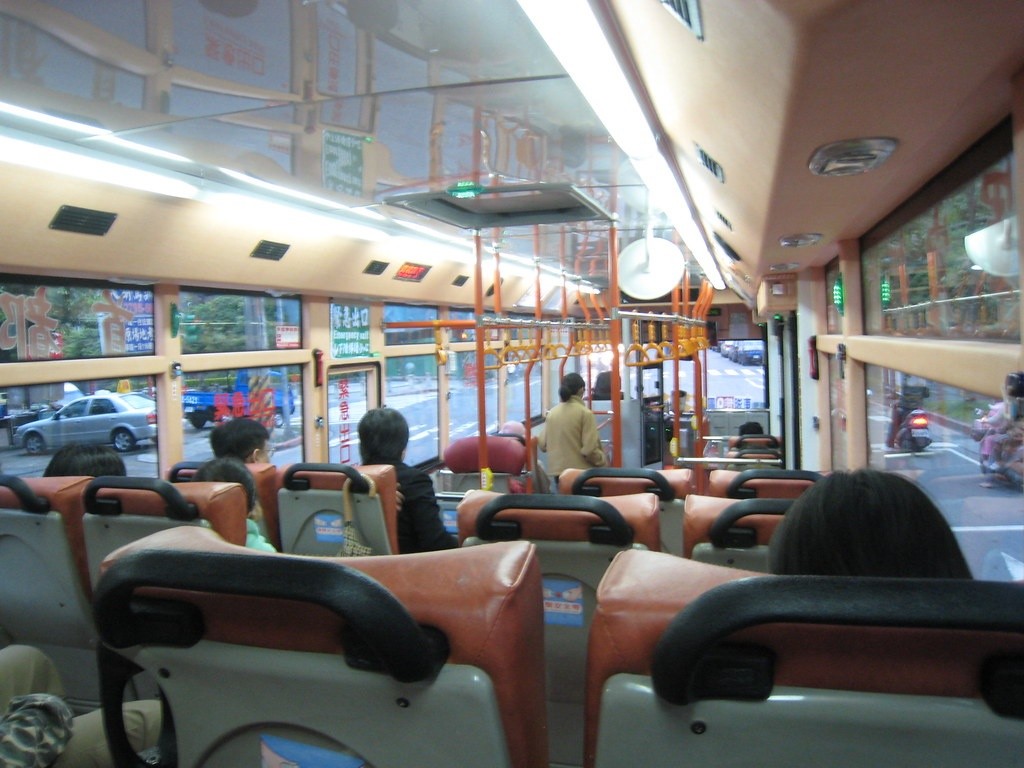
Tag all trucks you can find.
[182,367,295,429]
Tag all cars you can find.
[713,340,765,365]
[13,390,157,454]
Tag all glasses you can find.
[261,447,276,457]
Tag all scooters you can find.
[884,386,932,454]
[972,409,1024,488]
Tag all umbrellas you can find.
[333,472,375,556]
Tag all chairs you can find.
[0,434,1024,768]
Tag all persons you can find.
[42,441,127,478]
[192,456,278,553]
[536,372,610,491]
[210,419,405,511]
[768,464,974,579]
[979,395,1024,489]
[358,408,460,556]
[497,420,551,494]
[885,368,930,448]
[0,644,162,768]
